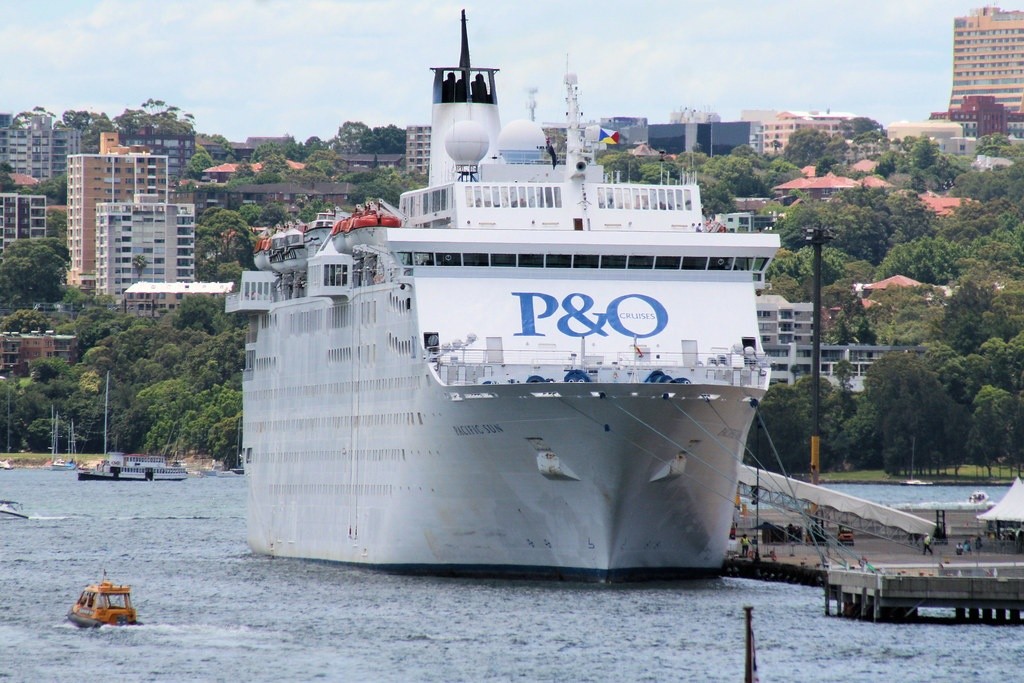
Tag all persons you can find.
[706,220,711,225]
[922,533,933,555]
[997,532,1015,546]
[212,458,216,468]
[696,223,703,232]
[973,492,983,502]
[956,537,982,555]
[740,534,752,556]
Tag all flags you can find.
[599,129,619,144]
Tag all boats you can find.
[199,463,221,477]
[66,570,143,631]
[343,203,402,250]
[332,216,353,255]
[78,370,188,482]
[252,224,308,274]
[0,500,28,521]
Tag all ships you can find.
[224,8,784,584]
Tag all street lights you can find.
[805,224,837,535]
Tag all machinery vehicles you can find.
[837,521,856,547]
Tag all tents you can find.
[976,476,1024,541]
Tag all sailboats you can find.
[900,435,934,486]
[42,404,78,470]
[0,386,15,470]
[215,416,244,477]
